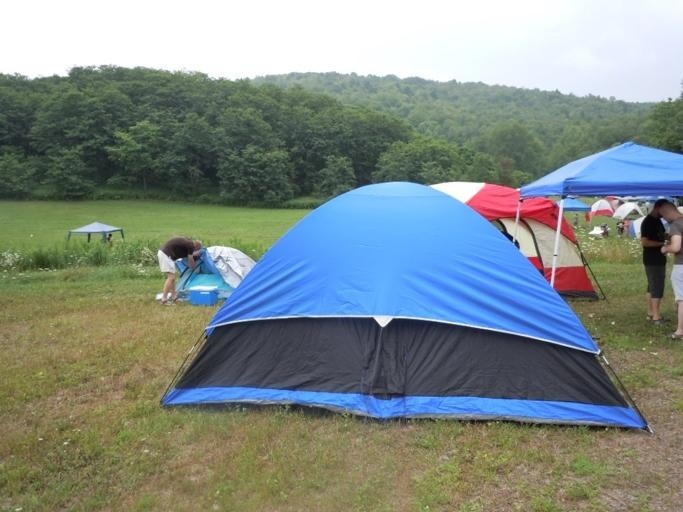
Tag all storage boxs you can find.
[189,286,219,305]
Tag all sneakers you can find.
[645,313,672,325]
[666,327,683,340]
[160,297,182,306]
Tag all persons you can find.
[155,235,204,308]
[642,198,670,324]
[106,234,114,244]
[572,213,579,227]
[585,208,591,224]
[656,200,682,340]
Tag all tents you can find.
[147,178,651,432]
[421,180,598,302]
[162,245,254,304]
[591,196,682,240]
